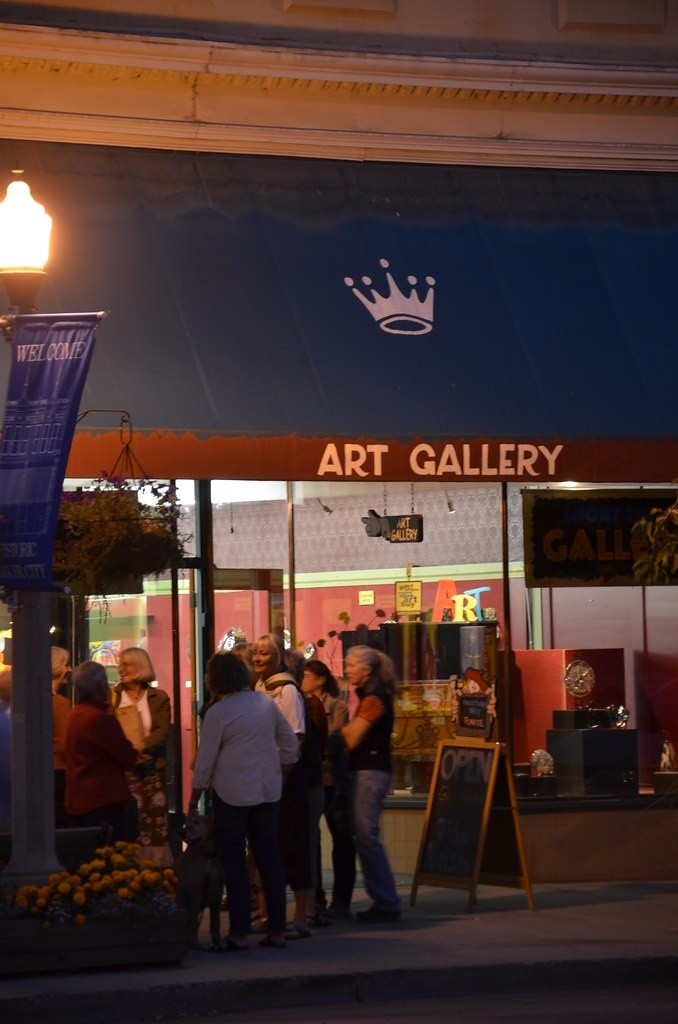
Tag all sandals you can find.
[306,913,333,927]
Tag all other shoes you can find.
[251,915,270,933]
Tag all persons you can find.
[181,634,358,945]
[63,661,141,843]
[51,646,72,830]
[186,651,299,948]
[109,647,174,871]
[0,664,12,832]
[328,645,401,925]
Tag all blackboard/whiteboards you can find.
[412,738,502,890]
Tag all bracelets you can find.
[189,803,198,809]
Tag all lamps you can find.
[0,170,54,275]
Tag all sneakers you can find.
[328,902,351,919]
[356,905,402,923]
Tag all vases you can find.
[85,516,180,580]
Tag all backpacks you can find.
[269,680,329,765]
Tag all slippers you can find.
[287,920,312,939]
[204,937,248,952]
[260,934,287,949]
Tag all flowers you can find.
[61,470,194,625]
